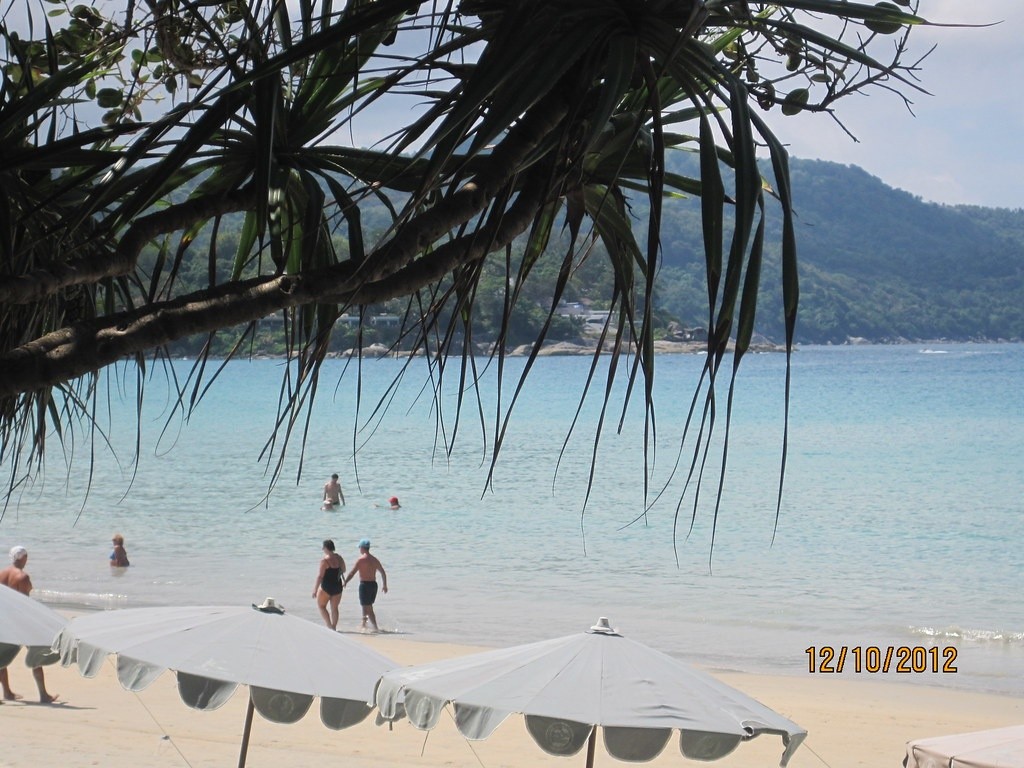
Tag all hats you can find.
[389,497,398,502]
[9,546,27,563]
[358,540,370,547]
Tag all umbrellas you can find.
[1,583,81,672]
[366,614,809,766]
[49,599,410,767]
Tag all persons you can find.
[322,497,335,511]
[387,495,401,511]
[343,538,389,633]
[321,473,347,505]
[311,539,346,632]
[108,533,131,567]
[0,546,60,704]
